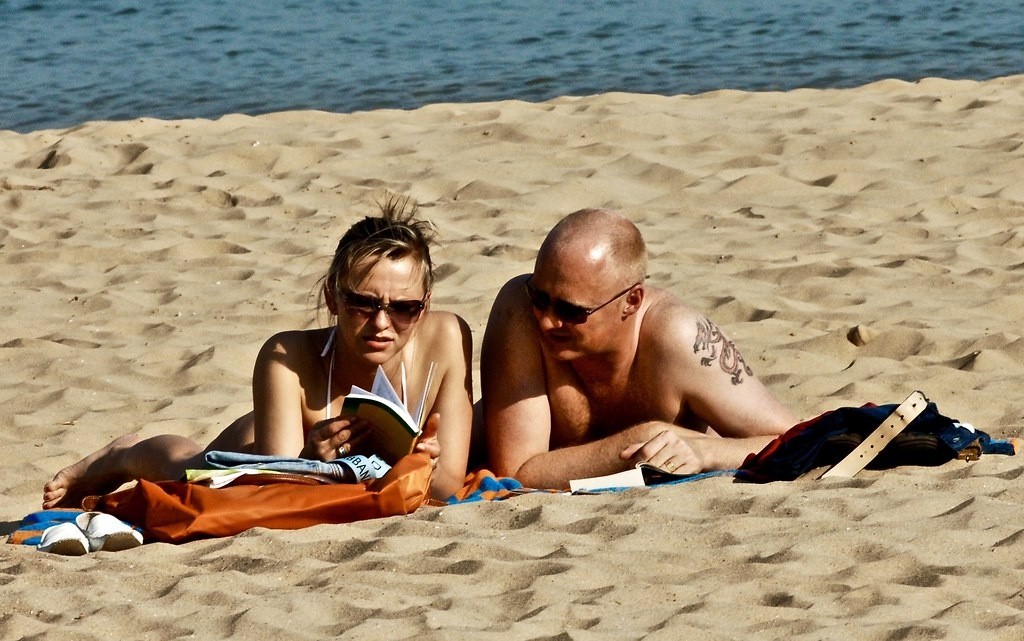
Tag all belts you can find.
[817,390,929,482]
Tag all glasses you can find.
[336,274,427,324]
[524,276,642,324]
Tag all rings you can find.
[664,460,677,472]
[339,447,350,456]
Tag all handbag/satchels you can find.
[81,450,437,545]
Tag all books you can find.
[569,460,698,495]
[340,361,439,468]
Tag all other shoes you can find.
[37,521,89,556]
[819,433,862,459]
[75,511,144,555]
[877,433,938,461]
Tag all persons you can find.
[44,191,473,509]
[466,208,802,489]
